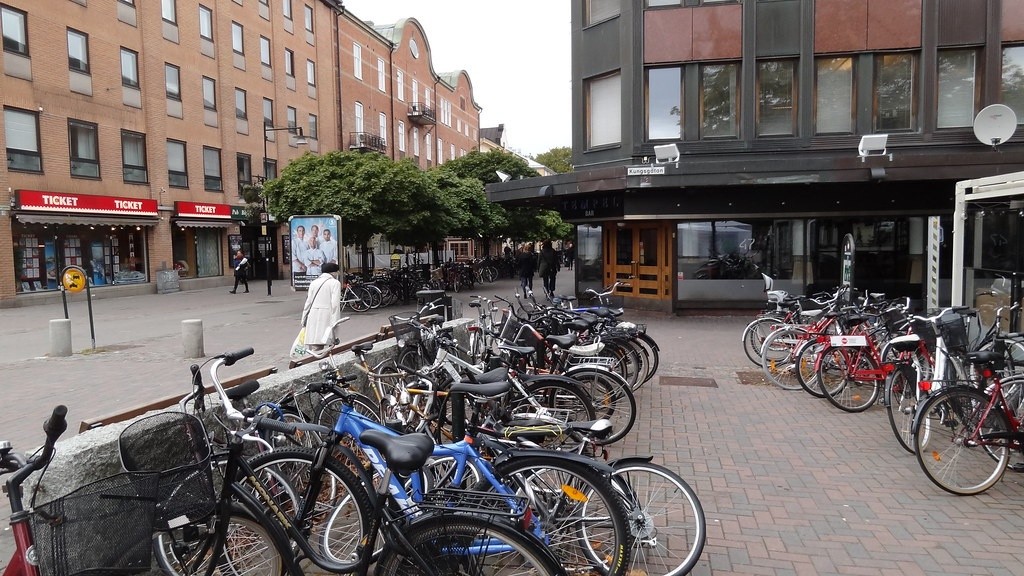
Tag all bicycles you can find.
[0,254,706,576]
[740,282,1024,496]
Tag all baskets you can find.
[117,411,218,529]
[388,310,420,343]
[28,470,160,576]
[517,305,536,327]
[911,308,969,354]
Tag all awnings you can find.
[175,221,233,228]
[16,214,159,228]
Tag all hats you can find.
[322,263,339,273]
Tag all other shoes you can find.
[229,291,236,294]
[243,291,249,293]
[550,293,554,298]
[524,293,527,299]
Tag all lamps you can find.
[654,144,680,164]
[539,185,553,197]
[858,133,889,157]
[496,171,512,183]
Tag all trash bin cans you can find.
[416,290,446,321]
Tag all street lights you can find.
[263,121,308,296]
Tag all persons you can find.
[292,225,310,276]
[517,244,536,299]
[566,246,574,270]
[301,262,342,351]
[504,245,511,258]
[228,250,251,293]
[304,237,326,276]
[306,225,324,250]
[319,229,338,266]
[537,241,560,297]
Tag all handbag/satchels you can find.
[539,263,549,278]
[564,261,569,267]
[290,326,324,364]
